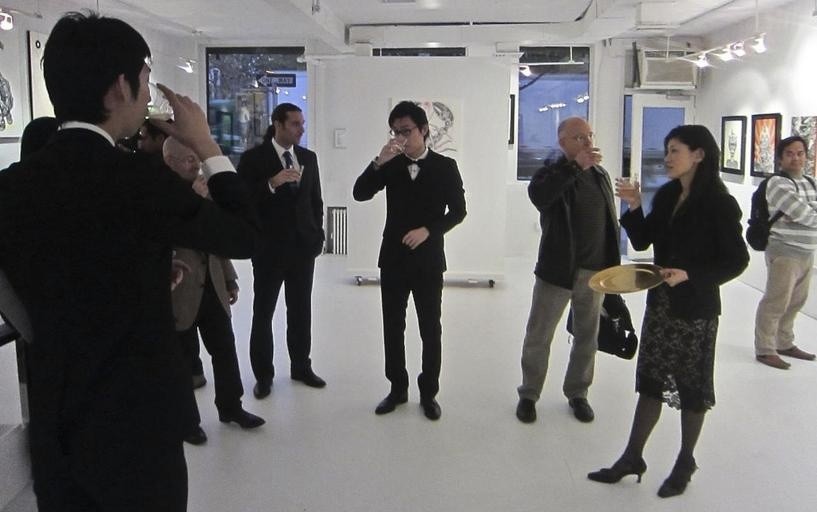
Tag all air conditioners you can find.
[638,50,700,91]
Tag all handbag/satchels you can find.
[565,293,638,359]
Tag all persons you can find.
[21,118,266,446]
[0,12,260,512]
[517,117,624,421]
[352,99,466,421]
[589,125,750,495]
[236,104,326,397]
[755,136,815,369]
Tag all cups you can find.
[145,81,173,122]
[289,164,304,178]
[393,136,408,154]
[620,177,637,199]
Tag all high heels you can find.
[658,457,696,497]
[588,458,646,483]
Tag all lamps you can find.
[0,0,43,32]
[664,0,768,70]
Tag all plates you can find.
[587,263,669,294]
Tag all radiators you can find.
[330,208,348,256]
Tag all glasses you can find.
[576,134,595,142]
[389,125,417,136]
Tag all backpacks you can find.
[746,171,817,251]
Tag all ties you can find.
[283,152,297,192]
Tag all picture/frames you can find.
[749,113,780,179]
[719,116,747,175]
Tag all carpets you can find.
[738,240,817,320]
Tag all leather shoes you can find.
[420,394,441,419]
[291,368,326,387]
[184,426,207,445]
[219,411,265,427]
[374,389,408,415]
[516,399,537,423]
[777,345,816,360]
[756,354,790,370]
[568,397,594,422]
[254,374,273,398]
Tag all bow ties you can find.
[404,158,425,167]
